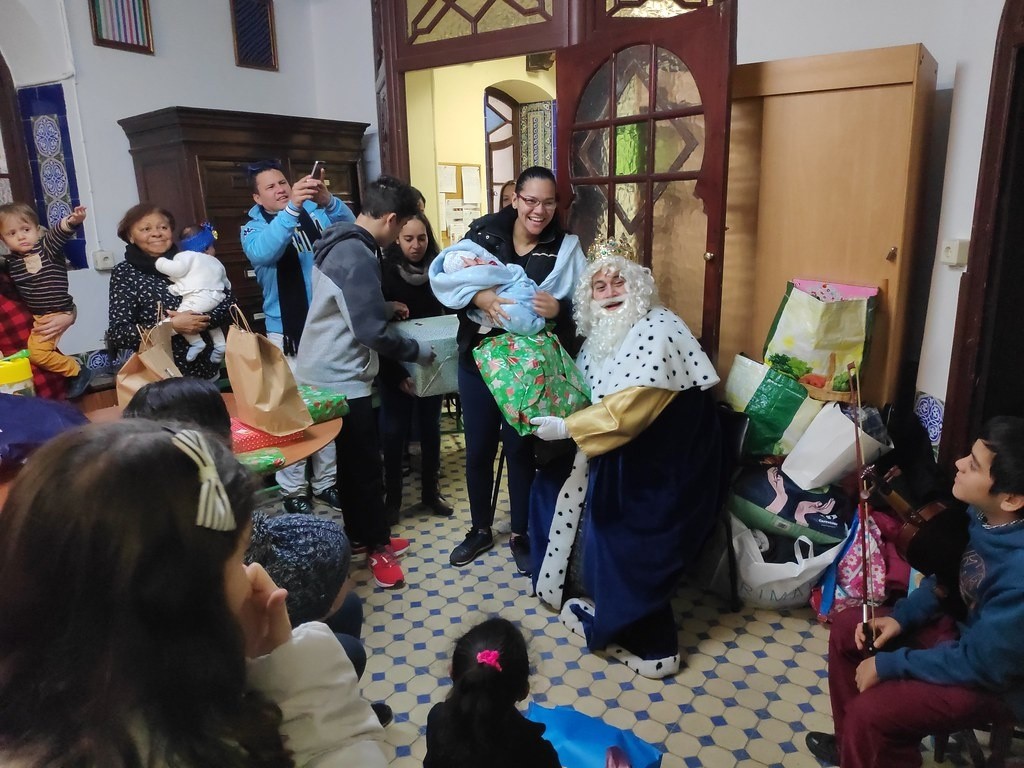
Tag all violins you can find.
[858,463,974,596]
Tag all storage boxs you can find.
[389,314,459,398]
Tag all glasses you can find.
[247,158,281,179]
[517,193,556,211]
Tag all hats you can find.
[241,510,350,628]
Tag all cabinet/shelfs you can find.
[116,105,370,331]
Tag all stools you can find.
[932,712,1023,768]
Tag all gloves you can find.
[529,415,572,442]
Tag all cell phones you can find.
[310,161,326,180]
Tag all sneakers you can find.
[311,485,342,512]
[350,536,410,561]
[449,526,494,566]
[509,534,531,575]
[363,545,405,588]
[282,496,311,515]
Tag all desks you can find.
[80,390,343,496]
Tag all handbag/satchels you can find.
[781,401,894,491]
[724,352,823,458]
[116,300,314,437]
[812,516,926,623]
[708,502,856,610]
[719,402,750,473]
[762,280,882,400]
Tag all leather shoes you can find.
[422,498,454,516]
[805,731,840,765]
[384,498,401,525]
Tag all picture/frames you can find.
[87,0,154,55]
[228,0,279,73]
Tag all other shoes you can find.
[369,702,393,728]
[67,365,92,398]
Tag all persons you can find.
[240,158,357,514]
[449,165,586,573]
[429,240,545,335]
[381,212,455,525]
[805,413,1024,768]
[103,202,240,384]
[244,510,393,728]
[394,186,440,476]
[500,181,518,207]
[295,174,436,588]
[529,232,720,677]
[0,417,392,768]
[0,201,96,398]
[424,616,561,768]
[122,377,235,453]
[154,222,231,363]
